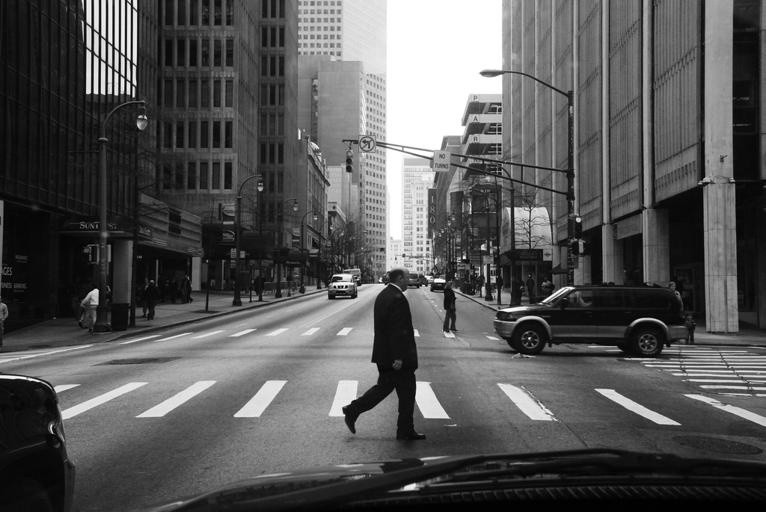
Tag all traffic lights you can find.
[574,216,582,238]
[345,148,354,174]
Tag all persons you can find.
[0,295,9,346]
[516,274,525,305]
[526,274,535,305]
[668,282,684,313]
[478,271,485,290]
[79,273,193,336]
[254,273,265,296]
[342,267,426,441]
[541,276,555,296]
[443,276,457,332]
[684,313,697,345]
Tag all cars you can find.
[0,371,77,511]
[408,271,446,292]
[378,271,391,284]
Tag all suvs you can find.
[492,285,692,358]
[328,273,358,300]
[342,268,362,286]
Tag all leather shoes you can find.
[340,404,359,435]
[395,429,426,444]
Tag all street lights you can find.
[277,197,299,300]
[330,227,345,276]
[232,173,264,306]
[477,69,577,287]
[93,98,149,336]
[317,221,334,291]
[300,211,318,294]
[437,209,472,276]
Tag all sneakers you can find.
[441,328,459,334]
